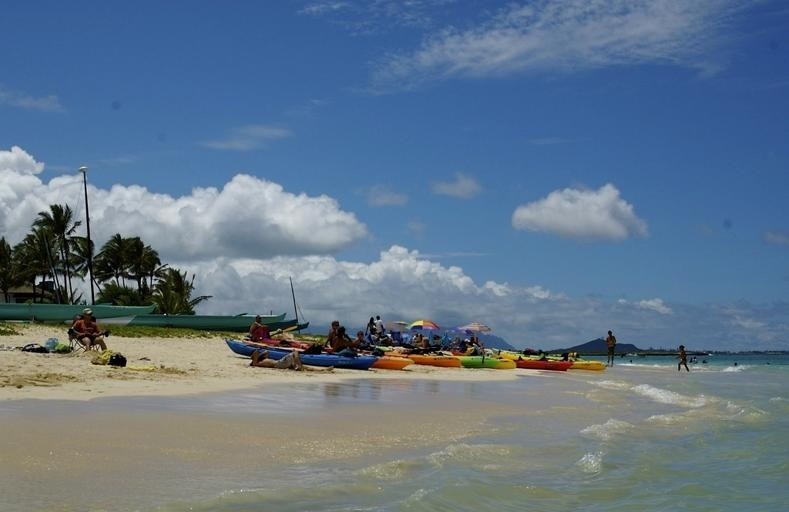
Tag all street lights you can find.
[79,164,97,306]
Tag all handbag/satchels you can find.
[92,349,126,366]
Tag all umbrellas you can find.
[393,321,408,332]
[409,318,439,335]
[458,322,491,341]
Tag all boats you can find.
[299,341,415,372]
[126,313,311,334]
[1,302,156,320]
[383,333,609,371]
[223,337,379,371]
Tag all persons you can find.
[412,332,486,356]
[324,319,340,347]
[332,326,357,350]
[249,315,271,341]
[536,349,546,360]
[250,348,334,372]
[674,344,690,372]
[365,316,376,336]
[69,308,107,351]
[374,315,385,341]
[605,329,617,368]
[353,330,369,348]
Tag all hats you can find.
[83,308,93,315]
